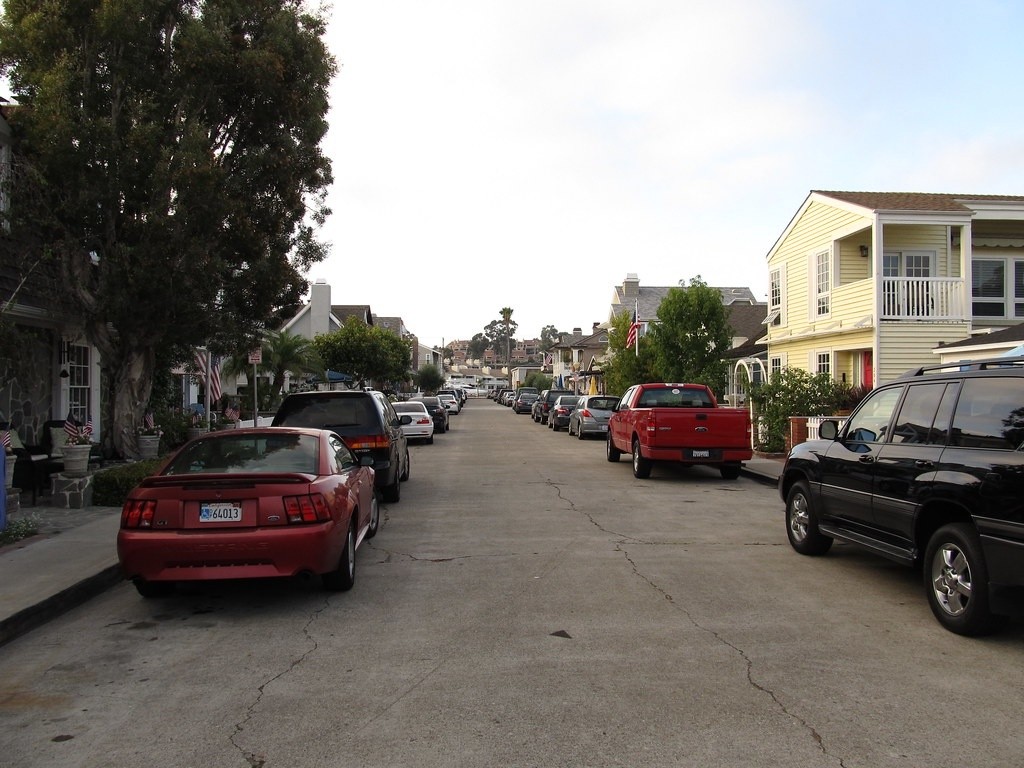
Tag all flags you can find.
[192,350,221,405]
[589,376,598,395]
[145,413,152,428]
[626,307,641,348]
[545,354,552,364]
[63,412,92,437]
[0,428,10,447]
[225,407,240,422]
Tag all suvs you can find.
[778,353,1024,635]
[266,385,412,505]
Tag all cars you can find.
[389,400,434,443]
[569,395,623,440]
[408,397,450,434]
[514,394,540,415]
[435,388,469,410]
[434,393,460,414]
[531,390,576,426]
[546,395,584,432]
[486,386,539,410]
[117,427,381,595]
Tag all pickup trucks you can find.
[605,382,754,481]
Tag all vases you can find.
[5,456,18,490]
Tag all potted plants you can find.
[54,410,238,473]
